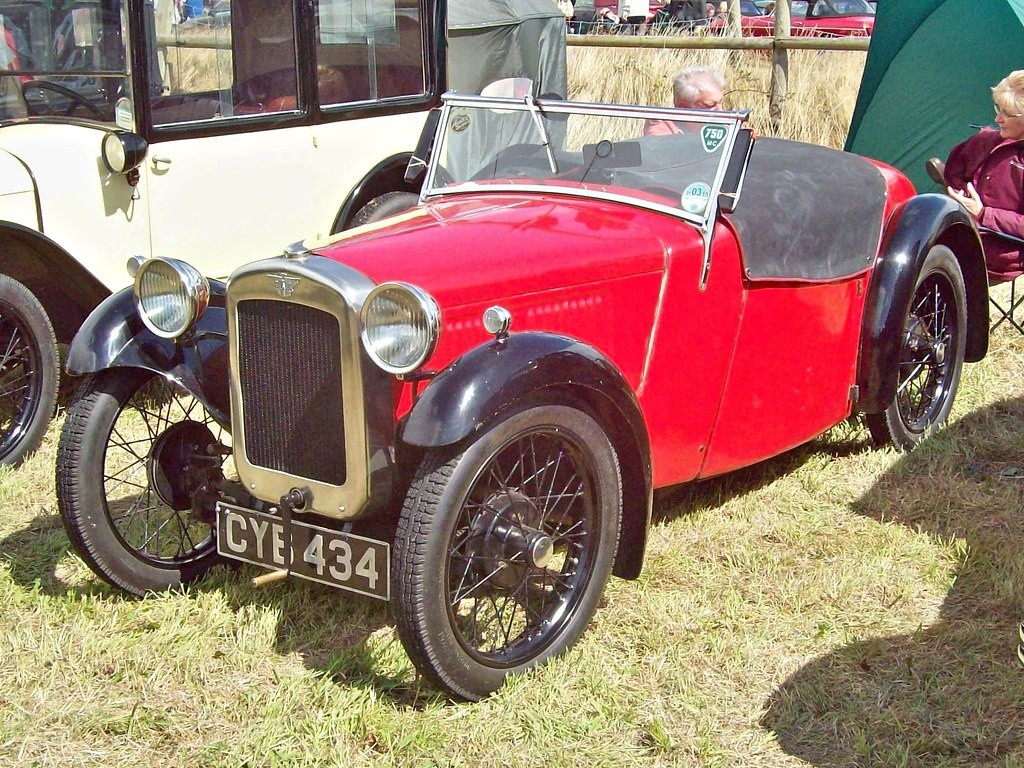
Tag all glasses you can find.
[993,104,1024,118]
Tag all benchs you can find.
[233,65,422,108]
[153,94,238,124]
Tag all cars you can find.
[55,91,990,708]
[749,0,878,43]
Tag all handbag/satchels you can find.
[557,0,574,19]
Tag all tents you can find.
[843,0,1024,193]
[320,0,568,180]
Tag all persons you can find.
[719,2,727,13]
[918,70,1024,287]
[706,2,715,17]
[766,2,776,15]
[644,68,742,135]
[566,15,579,33]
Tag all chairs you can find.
[819,4,832,16]
[978,225,1024,336]
